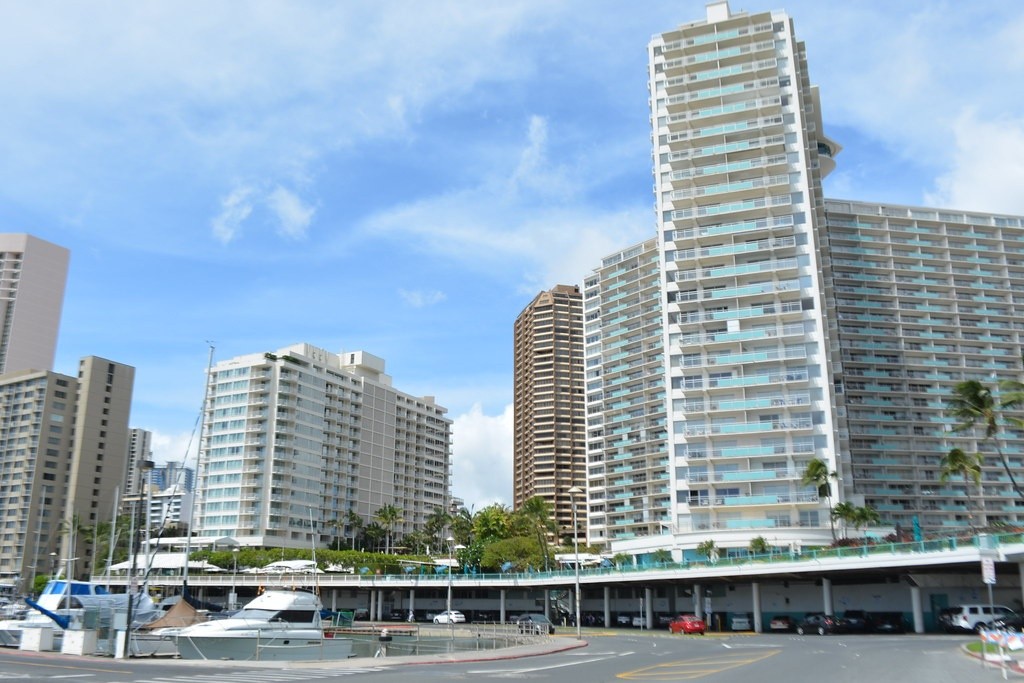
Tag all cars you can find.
[517,614,555,635]
[658,614,705,635]
[616,614,646,628]
[390,609,406,622]
[795,609,911,636]
[769,615,797,632]
[426,610,466,625]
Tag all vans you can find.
[951,604,1015,635]
[731,612,754,632]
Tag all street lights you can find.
[446,536,455,624]
[122,459,155,659]
[231,548,240,610]
[568,486,583,639]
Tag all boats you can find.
[161,590,354,660]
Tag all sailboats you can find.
[0,340,216,656]
